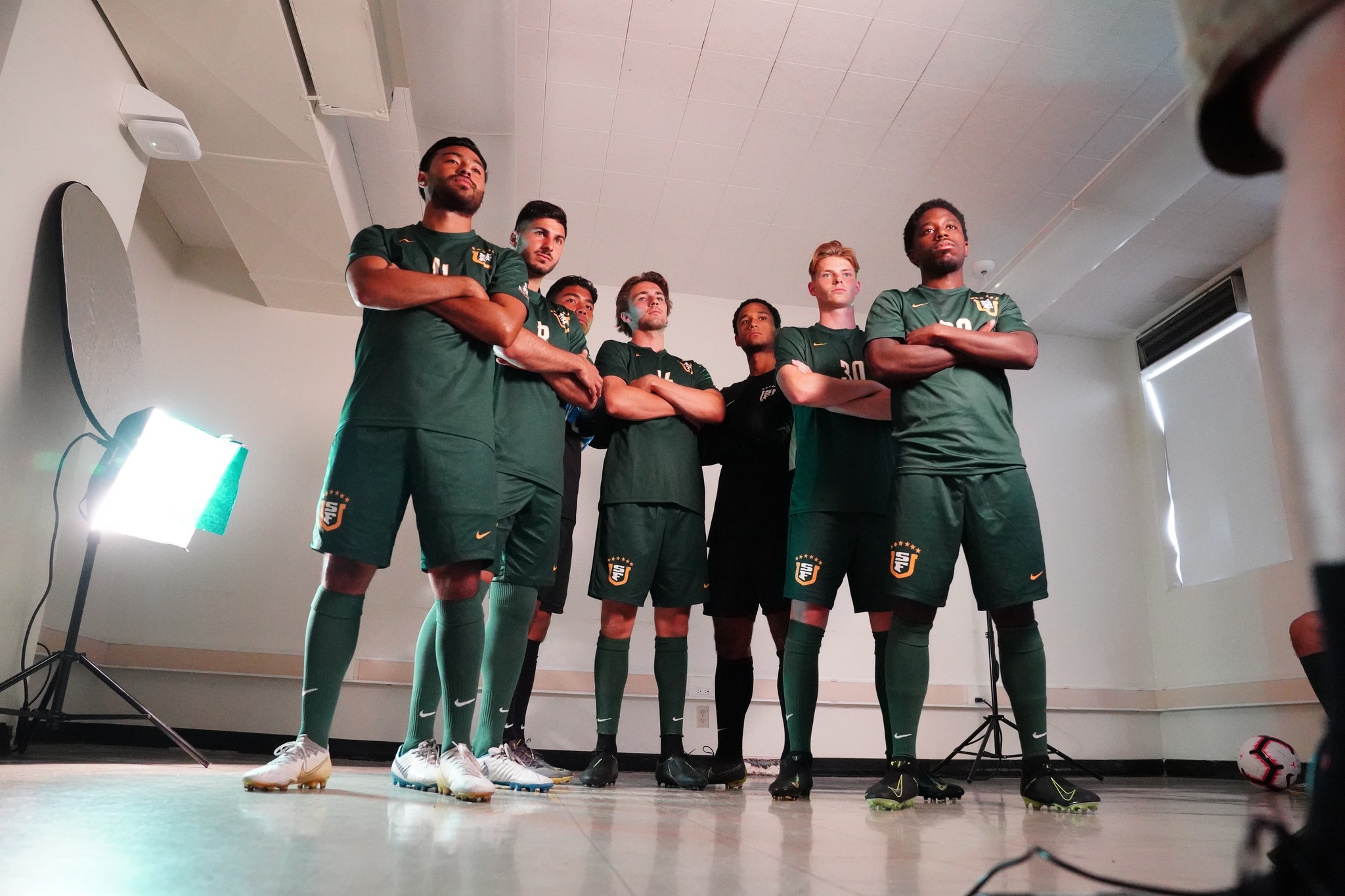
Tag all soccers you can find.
[1237,735,1301,792]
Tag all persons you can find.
[689,297,813,789]
[391,201,569,792]
[767,240,963,808]
[240,137,528,800]
[868,199,1101,814]
[501,274,602,786]
[576,270,726,787]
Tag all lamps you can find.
[0,399,258,771]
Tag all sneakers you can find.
[656,748,709,790]
[886,760,965,804]
[243,734,333,792]
[390,738,441,793]
[690,746,747,788]
[1017,755,1101,815]
[439,741,495,802]
[507,738,573,785]
[768,751,814,800]
[864,759,919,811]
[471,743,556,793]
[579,750,619,787]
